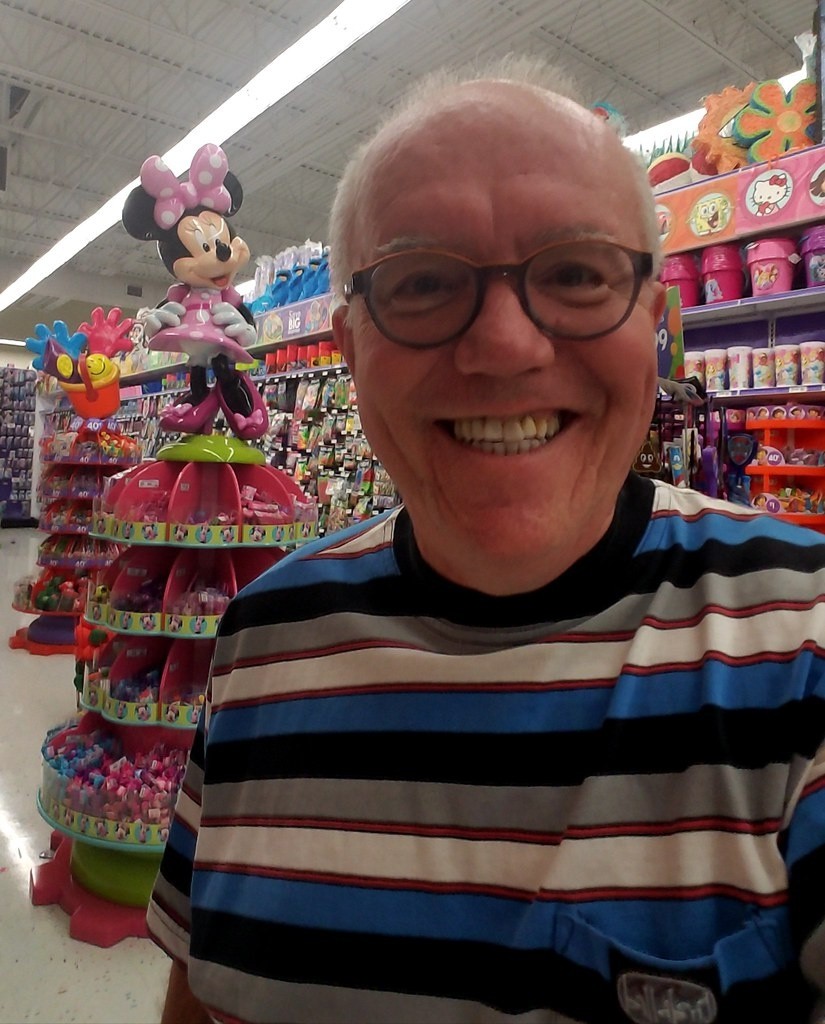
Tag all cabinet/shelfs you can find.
[30,143,319,949]
[1,146,824,660]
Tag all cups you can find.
[745,237,801,265]
[699,245,744,277]
[684,341,825,393]
[801,224,825,256]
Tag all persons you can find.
[145,55,825,1024]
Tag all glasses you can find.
[342,237,653,342]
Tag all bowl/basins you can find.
[658,253,700,285]
[662,278,701,307]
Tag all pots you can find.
[701,269,749,304]
[748,257,804,297]
[802,249,825,288]
